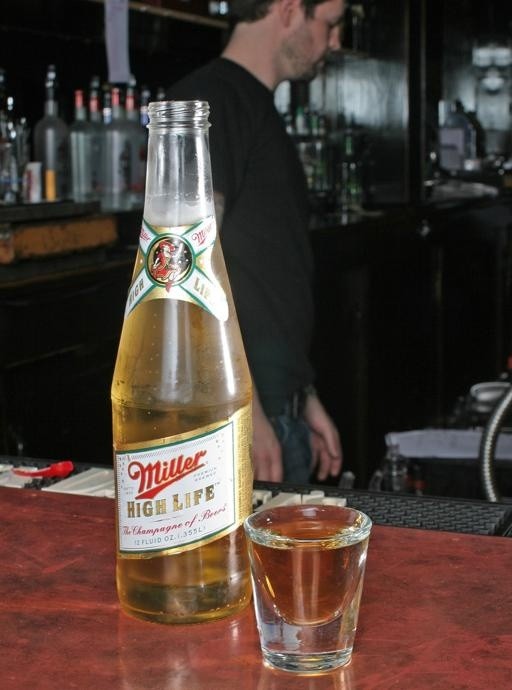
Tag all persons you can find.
[163,0,348,483]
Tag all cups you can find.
[243,502,372,676]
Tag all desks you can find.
[0,488,512,690]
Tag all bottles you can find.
[110,98,255,626]
[426,93,509,205]
[371,435,417,494]
[275,101,372,217]
[1,65,175,219]
